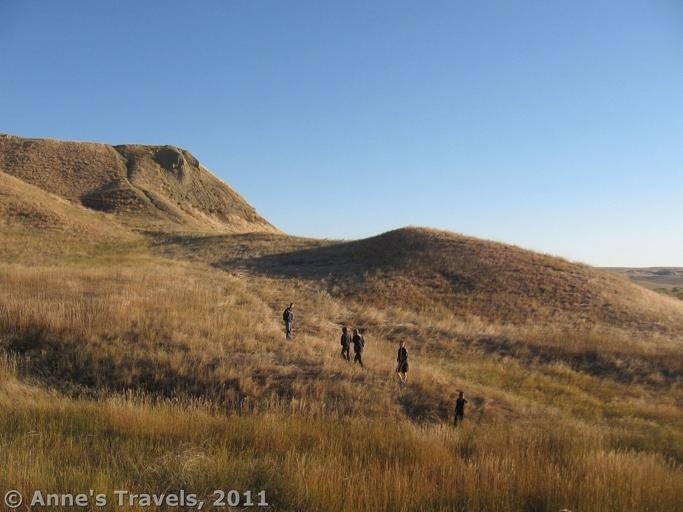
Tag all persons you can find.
[395,340,409,384]
[453,391,468,427]
[282,302,295,340]
[340,326,352,362]
[352,328,365,368]
